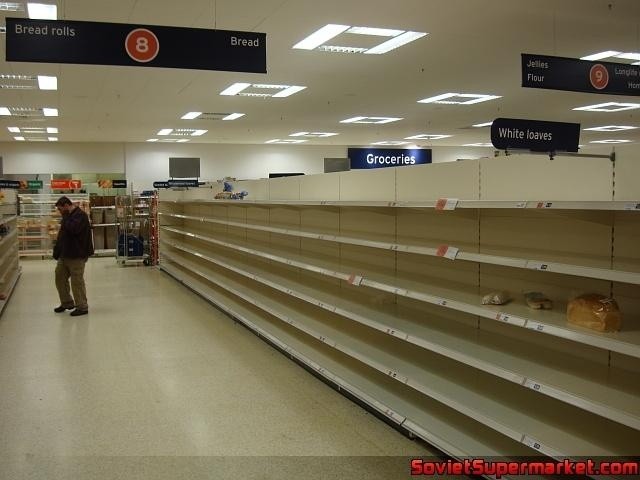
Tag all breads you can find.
[482,289,511,305]
[567,293,625,333]
[525,293,553,310]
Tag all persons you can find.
[51,195,96,318]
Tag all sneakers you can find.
[53,304,75,313]
[69,308,89,317]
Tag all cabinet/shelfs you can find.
[0,188,157,316]
[158,143,639,477]
